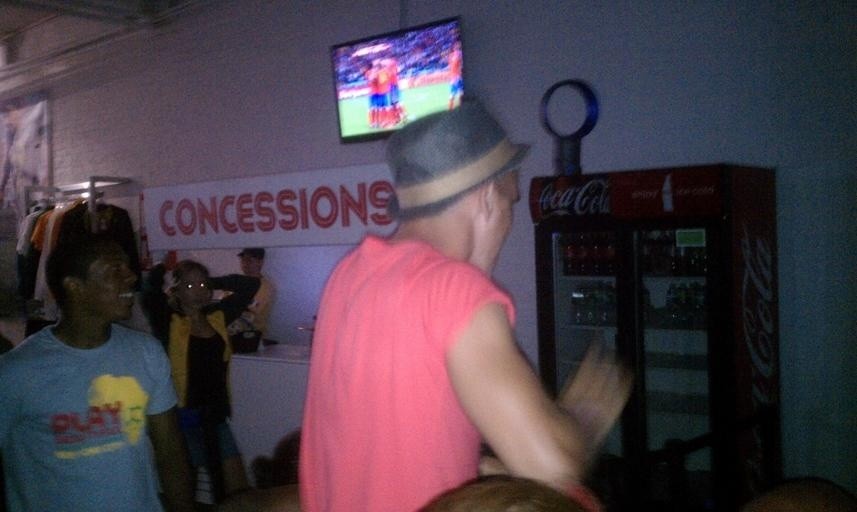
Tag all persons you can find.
[420,474,590,511]
[295,100,637,511]
[136,248,262,511]
[220,248,273,343]
[333,14,462,133]
[743,475,855,511]
[1,226,202,512]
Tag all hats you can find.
[385,102,532,219]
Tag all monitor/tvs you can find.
[331,16,465,143]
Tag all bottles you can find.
[564,229,707,326]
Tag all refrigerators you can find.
[529,161,786,499]
